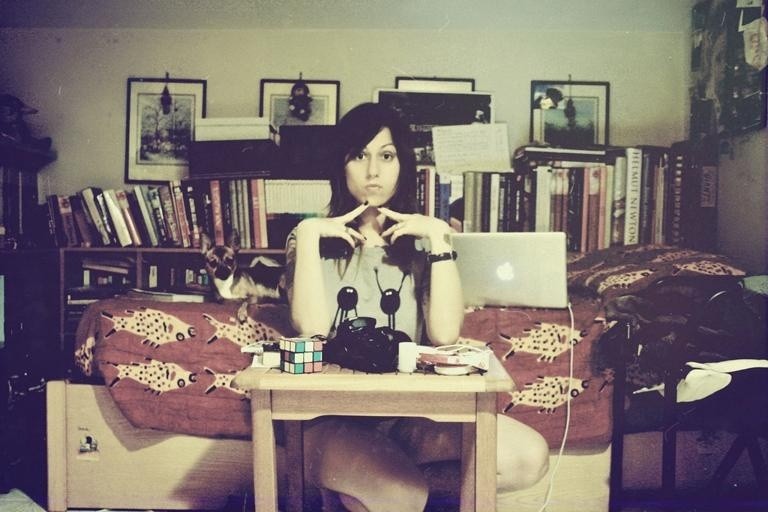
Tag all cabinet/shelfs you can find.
[0,130,57,495]
[57,245,286,375]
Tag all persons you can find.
[283,102,552,511]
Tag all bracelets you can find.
[425,247,458,264]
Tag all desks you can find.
[233,350,516,510]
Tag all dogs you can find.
[198,228,288,324]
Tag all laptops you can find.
[450,231,568,310]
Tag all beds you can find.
[44,244,768,510]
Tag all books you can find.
[48,175,332,298]
[413,140,717,256]
[418,345,486,371]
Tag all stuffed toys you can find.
[287,83,316,123]
